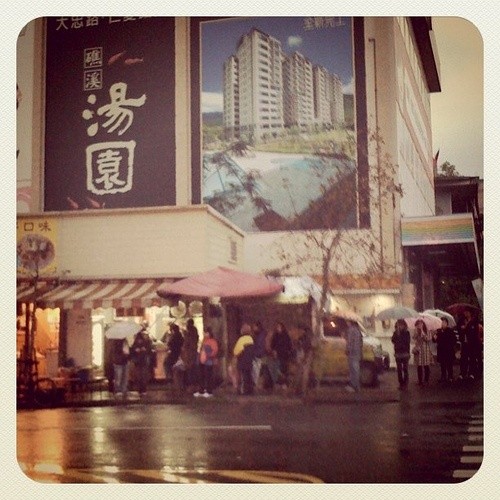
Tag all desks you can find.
[68,375,106,400]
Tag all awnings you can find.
[16,278,189,310]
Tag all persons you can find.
[343,318,361,392]
[412,319,434,387]
[264,322,319,390]
[129,328,158,395]
[455,306,479,380]
[432,317,457,382]
[163,321,186,396]
[109,338,130,397]
[229,322,267,395]
[391,318,410,390]
[182,317,198,385]
[192,326,219,396]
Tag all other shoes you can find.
[130,390,139,397]
[345,385,356,392]
[193,392,213,398]
[116,392,123,400]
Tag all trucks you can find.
[222,301,391,389]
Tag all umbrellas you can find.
[105,322,144,339]
[446,303,482,320]
[375,306,420,334]
[332,309,365,322]
[425,309,457,328]
[405,312,443,331]
[159,268,287,375]
